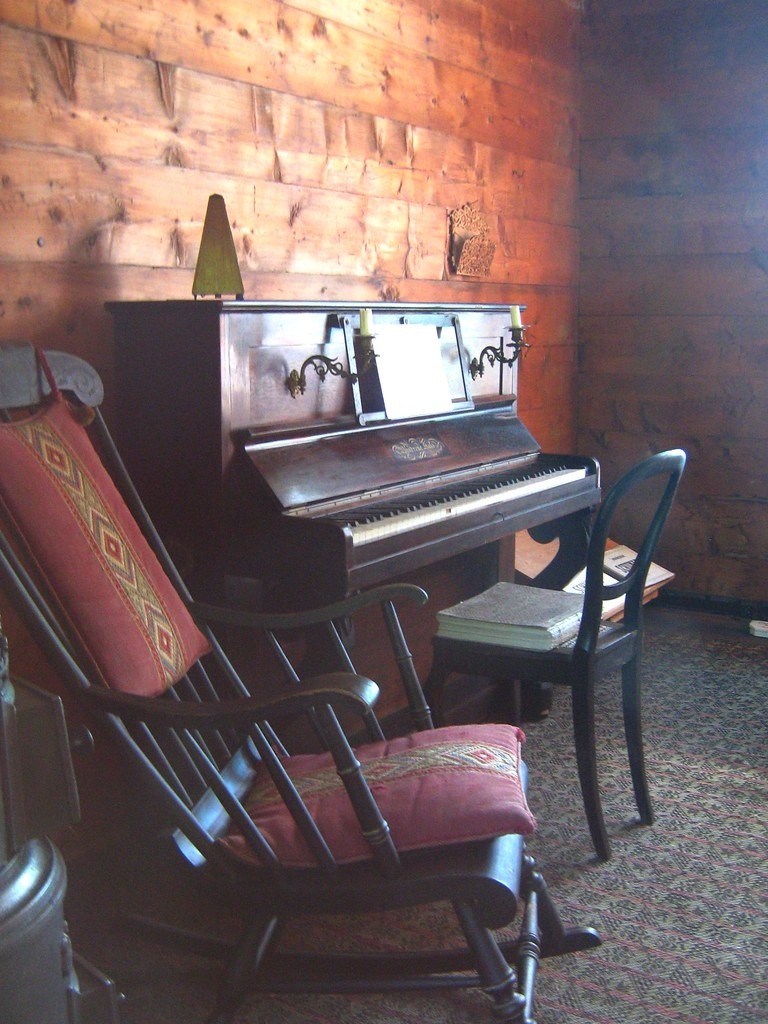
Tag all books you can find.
[436,582,584,652]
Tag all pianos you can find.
[102,296,600,740]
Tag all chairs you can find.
[0,349,601,1024]
[428,447,687,862]
